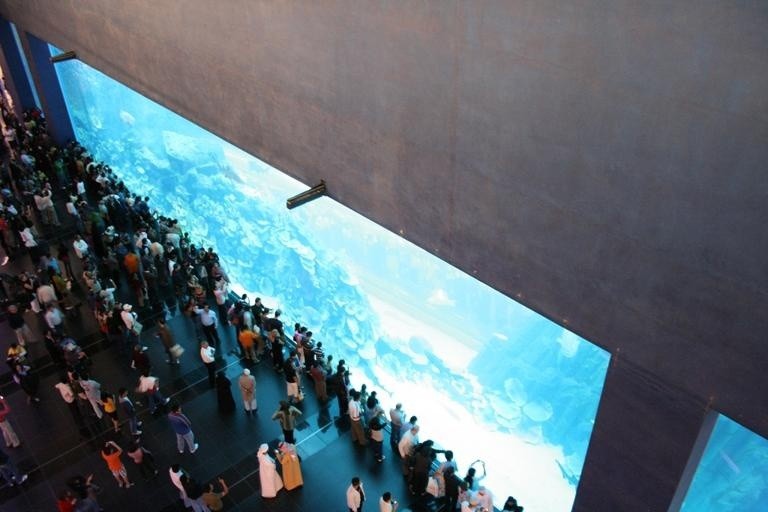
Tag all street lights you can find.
[169,344,184,358]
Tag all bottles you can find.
[123,304,132,310]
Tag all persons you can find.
[3,104,522,511]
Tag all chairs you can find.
[131,430,142,435]
[163,397,170,405]
[191,443,198,453]
[10,474,28,487]
[378,456,385,462]
